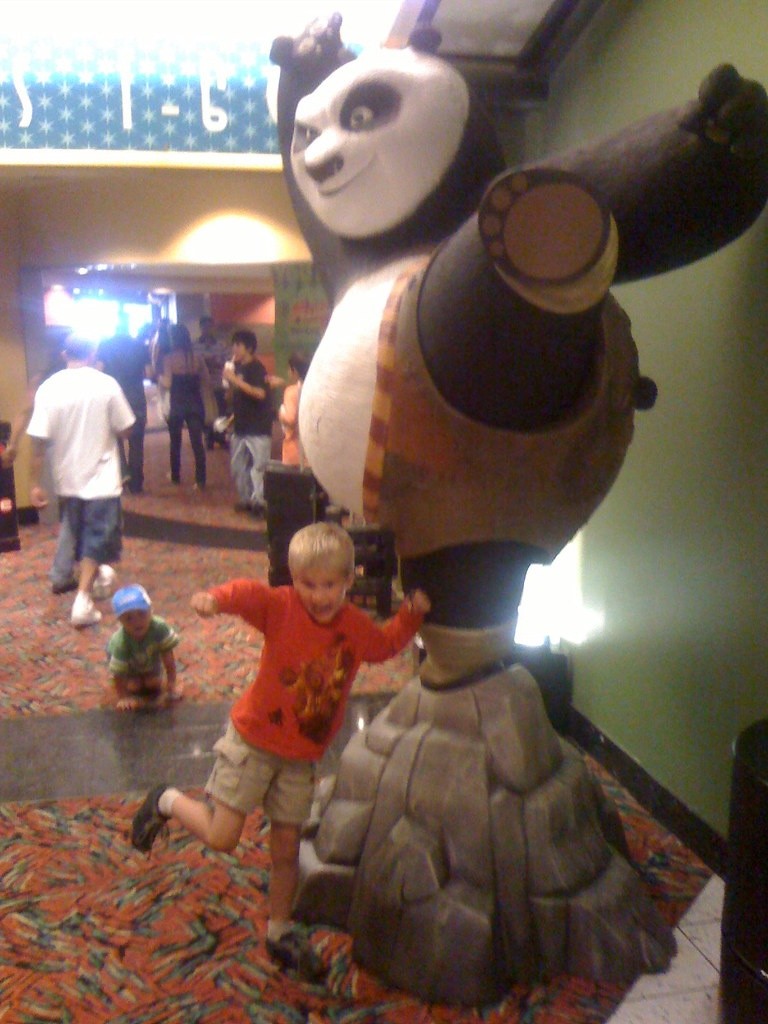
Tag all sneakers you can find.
[131,784,170,853]
[265,930,328,986]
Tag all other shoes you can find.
[71,592,102,626]
[93,564,117,598]
[234,503,267,520]
[52,579,79,594]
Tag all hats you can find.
[112,584,152,620]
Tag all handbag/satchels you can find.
[198,356,219,428]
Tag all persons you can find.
[130,522,432,983]
[27,335,138,626]
[93,311,268,514]
[279,350,317,465]
[105,583,179,710]
[3,340,78,595]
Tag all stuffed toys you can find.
[270,16,767,691]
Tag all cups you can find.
[223,361,236,389]
